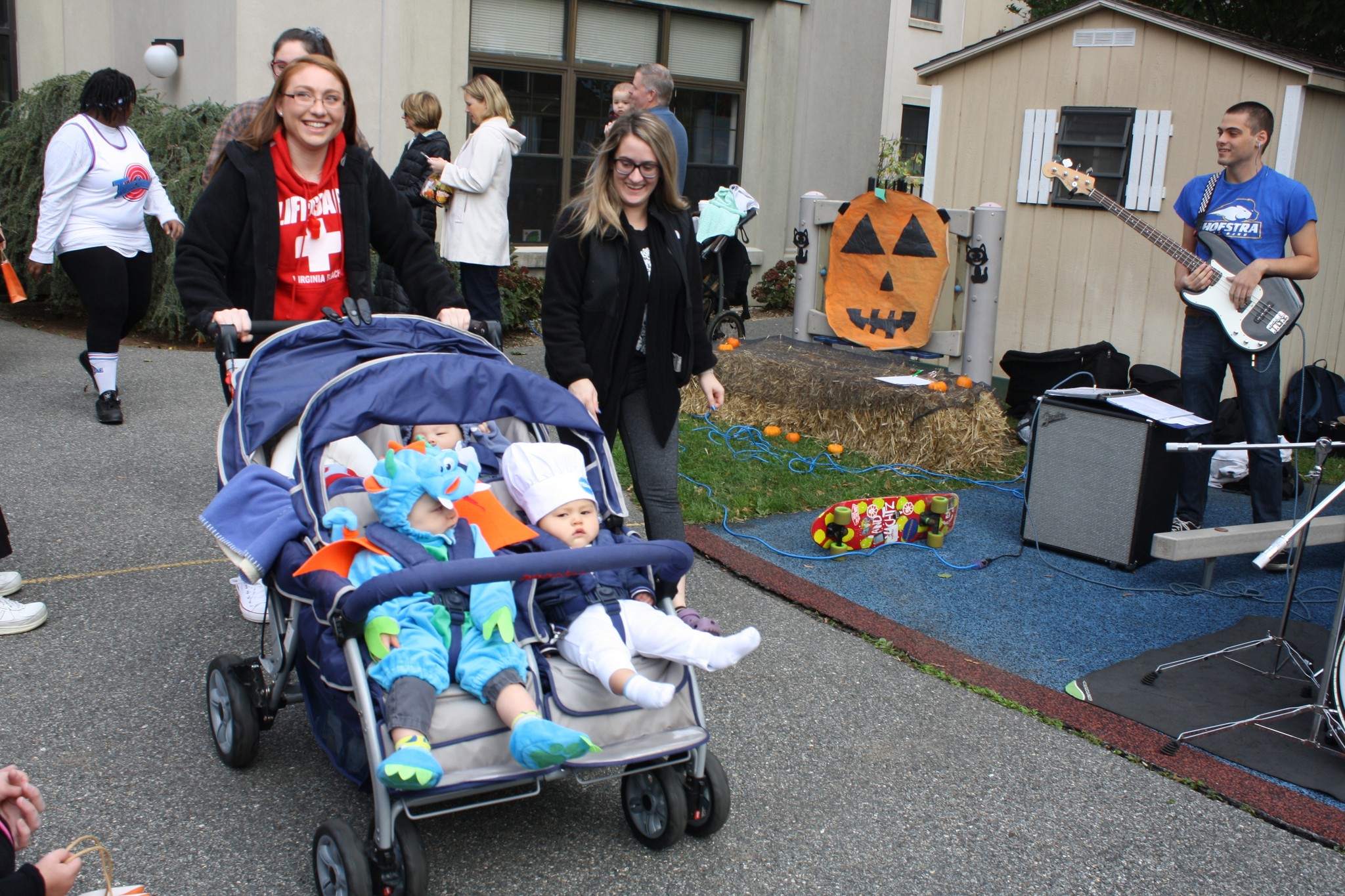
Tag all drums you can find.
[1332,629,1345,730]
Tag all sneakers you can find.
[0,572,22,596]
[0,596,48,635]
[1259,549,1293,570]
[96,390,123,422]
[79,350,118,396]
[229,574,269,624]
[1170,515,1202,533]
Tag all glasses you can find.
[279,90,346,109]
[271,60,287,77]
[613,158,661,179]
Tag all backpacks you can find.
[1209,435,1304,500]
[1282,359,1345,443]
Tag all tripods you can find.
[1144,436,1345,762]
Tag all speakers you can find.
[1020,395,1209,571]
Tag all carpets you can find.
[1064,612,1345,803]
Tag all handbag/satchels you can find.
[61,835,150,896]
[0,249,28,304]
[420,173,453,210]
[1000,340,1130,418]
[721,237,751,306]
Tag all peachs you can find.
[422,188,447,203]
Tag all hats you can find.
[501,441,598,526]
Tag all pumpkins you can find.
[928,381,947,392]
[827,443,843,453]
[718,342,733,351]
[727,333,739,348]
[764,425,781,437]
[786,428,800,443]
[956,373,973,388]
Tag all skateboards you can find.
[809,493,958,562]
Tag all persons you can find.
[1171,101,1318,574]
[24,68,187,422]
[174,56,472,621]
[0,227,82,896]
[549,107,723,639]
[320,416,762,793]
[203,28,688,359]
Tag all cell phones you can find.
[420,151,432,160]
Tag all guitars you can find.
[1042,154,1304,351]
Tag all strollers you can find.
[689,207,757,347]
[204,320,732,896]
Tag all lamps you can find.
[141,35,185,83]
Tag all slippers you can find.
[677,609,721,636]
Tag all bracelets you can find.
[697,369,713,376]
